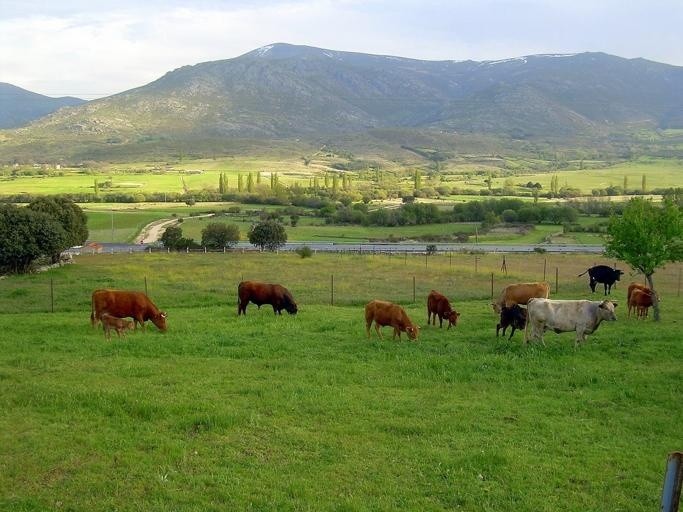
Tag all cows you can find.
[426,289,461,330]
[490,282,551,313]
[363,299,423,345]
[577,265,624,297]
[626,282,660,321]
[236,280,298,320]
[524,296,618,350]
[90,289,166,334]
[496,303,528,340]
[99,312,134,340]
